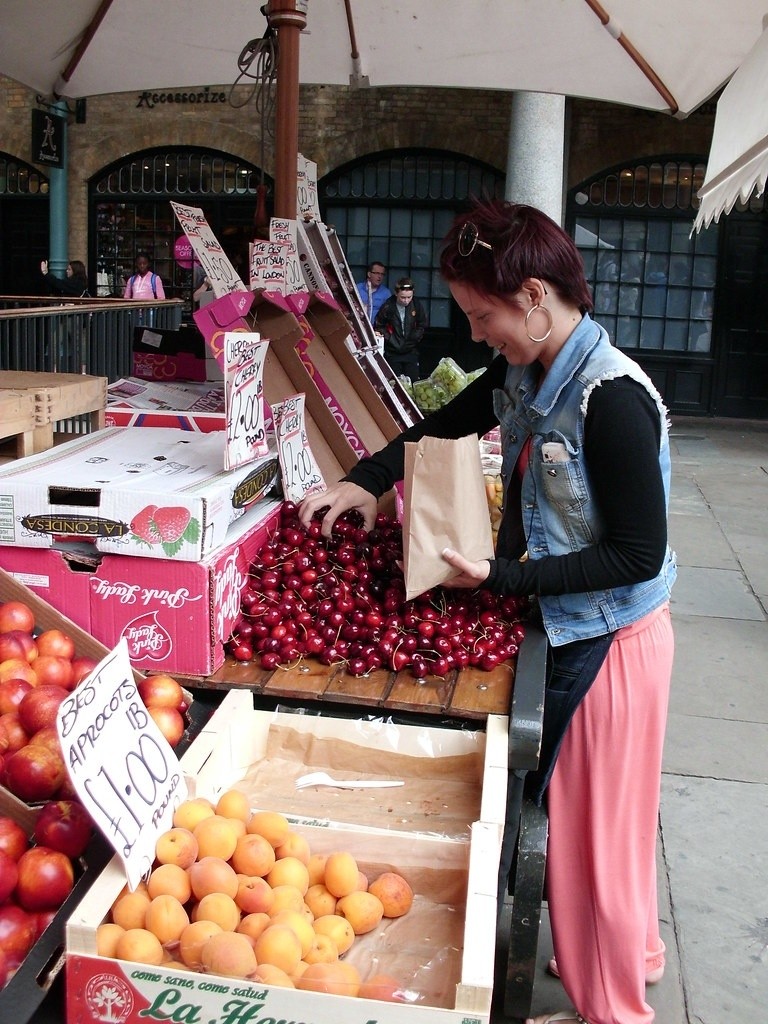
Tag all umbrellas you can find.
[0,0,768,218]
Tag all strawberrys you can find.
[131,505,191,543]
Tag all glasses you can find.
[370,271,386,277]
[397,284,414,291]
[457,223,548,296]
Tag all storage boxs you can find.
[0,219,510,1024]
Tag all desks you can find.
[137,528,552,1024]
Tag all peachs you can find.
[0,596,187,987]
[95,790,415,1000]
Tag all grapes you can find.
[394,360,488,411]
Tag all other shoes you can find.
[549,954,662,986]
[526,1006,589,1023]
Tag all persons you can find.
[123,255,166,327]
[356,261,392,327]
[373,276,427,384]
[40,259,90,374]
[601,258,712,352]
[296,187,673,1024]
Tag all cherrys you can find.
[223,502,527,677]
